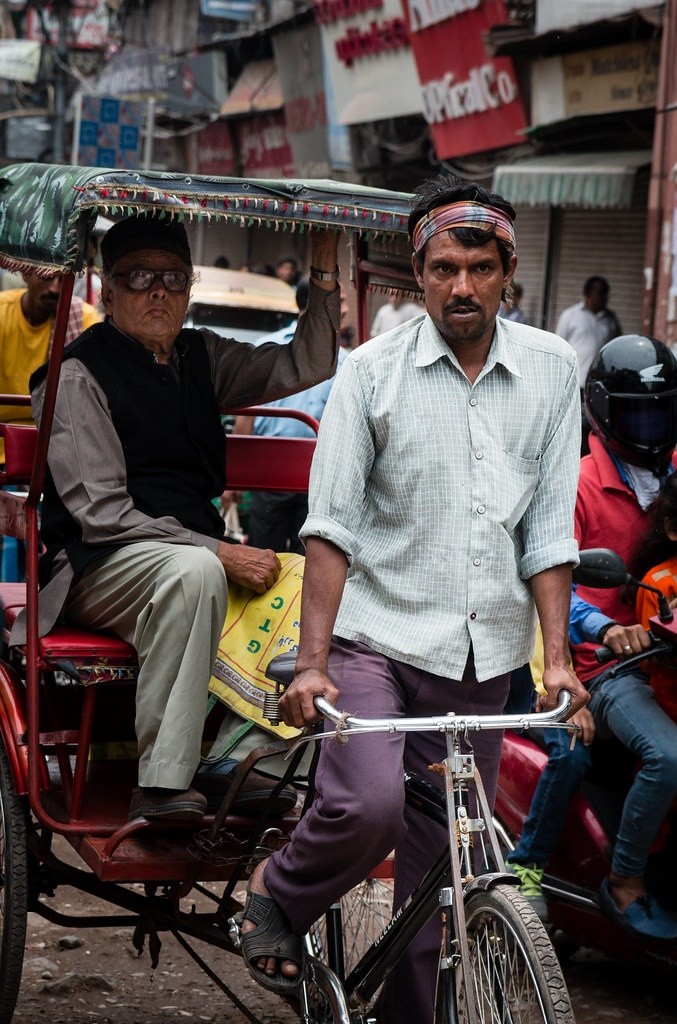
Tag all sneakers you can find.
[504,859,549,923]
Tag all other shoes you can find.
[195,763,299,818]
[128,783,208,825]
[597,877,677,941]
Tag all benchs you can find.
[0,395,319,670]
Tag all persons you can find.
[241,174,591,1024]
[507,623,592,919]
[28,209,341,819]
[569,335,677,938]
[554,275,622,458]
[219,275,350,557]
[369,288,422,339]
[498,283,525,323]
[0,269,103,583]
[214,254,297,283]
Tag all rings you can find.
[623,645,630,650]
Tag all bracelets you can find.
[597,623,621,645]
[310,265,340,281]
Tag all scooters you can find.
[493,545,677,997]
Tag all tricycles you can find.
[0,160,610,1024]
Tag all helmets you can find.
[585,335,677,473]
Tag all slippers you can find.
[242,877,308,990]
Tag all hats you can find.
[100,209,193,269]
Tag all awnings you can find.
[492,151,652,210]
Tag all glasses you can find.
[104,269,192,293]
